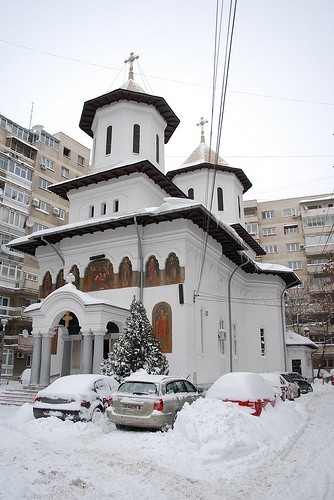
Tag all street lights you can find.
[1,319,8,332]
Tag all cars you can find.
[18,369,31,384]
[33,373,121,423]
[105,375,203,432]
[280,373,301,398]
[285,372,312,394]
[204,374,277,419]
[279,379,294,401]
